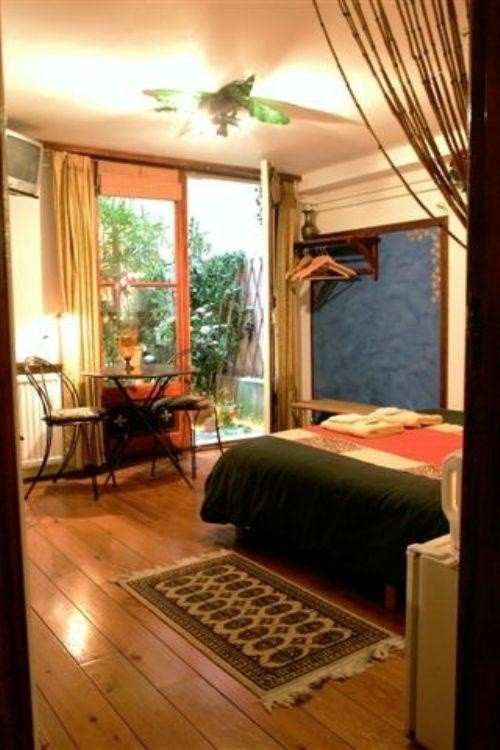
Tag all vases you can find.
[301,210,319,241]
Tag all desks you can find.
[80,366,203,498]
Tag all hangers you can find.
[284,247,357,284]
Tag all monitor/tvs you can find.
[5,128,44,198]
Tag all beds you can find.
[200,408,464,615]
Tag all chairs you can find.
[24,356,118,500]
[150,347,224,480]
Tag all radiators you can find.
[16,371,60,469]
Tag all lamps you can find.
[142,73,291,140]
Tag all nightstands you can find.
[401,526,454,749]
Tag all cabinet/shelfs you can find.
[16,364,76,478]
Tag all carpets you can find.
[108,548,405,715]
